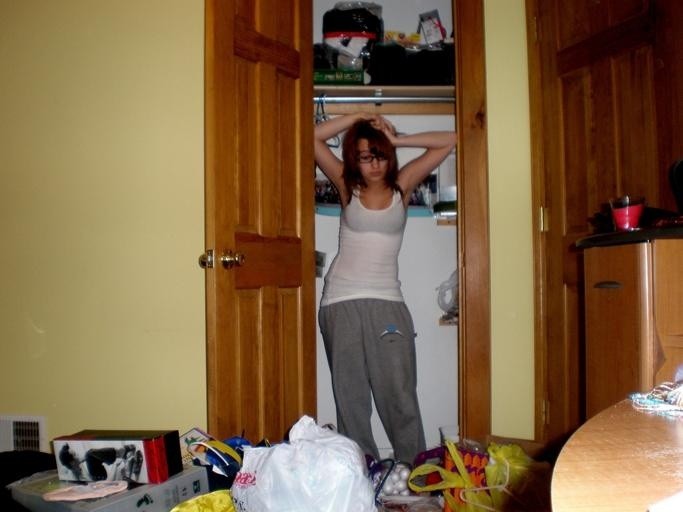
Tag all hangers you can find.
[313,92,341,148]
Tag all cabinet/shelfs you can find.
[569,229,682,426]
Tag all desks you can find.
[546,394,683,512]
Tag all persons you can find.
[312,109,458,464]
